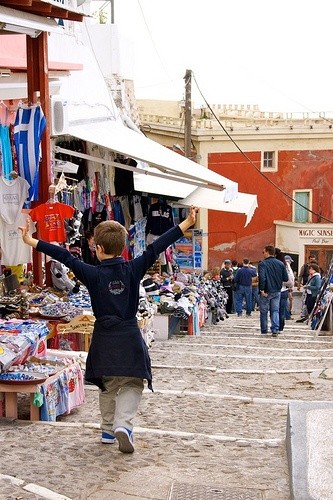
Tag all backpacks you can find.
[232,267,239,291]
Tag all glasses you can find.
[263,251,268,254]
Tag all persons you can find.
[219,249,328,332]
[18,206,198,454]
[257,246,288,337]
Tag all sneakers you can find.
[114,427,134,453]
[101,432,115,443]
[272,333,278,336]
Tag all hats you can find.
[284,255,294,262]
[224,260,231,264]
[142,279,158,292]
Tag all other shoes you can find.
[246,312,251,316]
[238,313,242,317]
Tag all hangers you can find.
[2,166,24,181]
[20,96,39,106]
[0,98,11,107]
[45,194,58,205]
